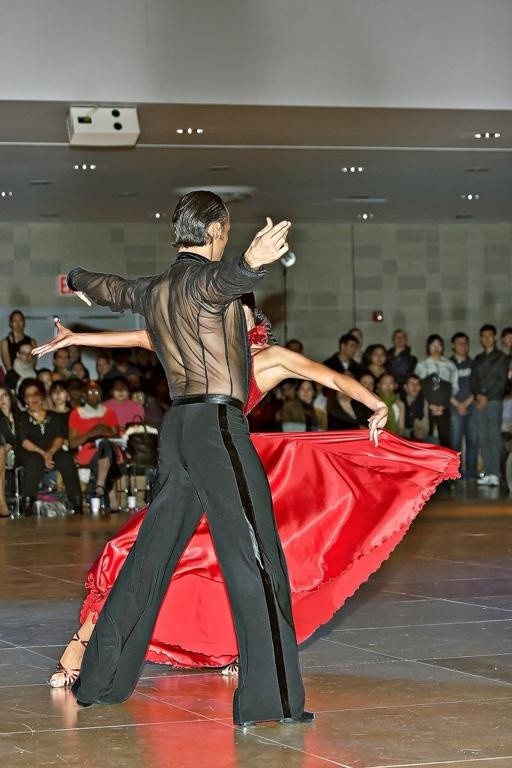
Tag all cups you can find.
[90,497,101,516]
[126,495,137,512]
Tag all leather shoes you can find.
[239,712,314,727]
[0,514,10,518]
[95,485,105,498]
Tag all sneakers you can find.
[477,474,500,486]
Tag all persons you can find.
[63,191,315,731]
[249,323,512,486]
[28,305,461,688]
[1,305,171,517]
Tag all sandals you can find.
[48,632,89,687]
[222,658,239,675]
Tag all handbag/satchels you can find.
[125,422,159,466]
